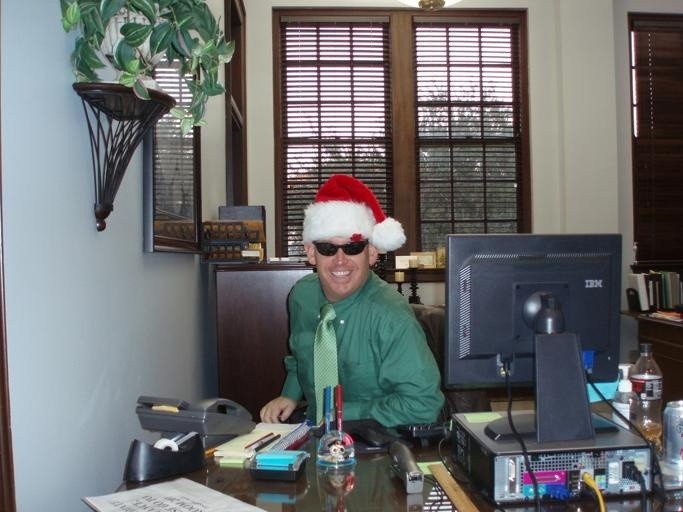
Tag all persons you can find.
[259,177,446,429]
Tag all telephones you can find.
[136,396,256,436]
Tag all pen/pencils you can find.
[335,384,343,445]
[325,385,331,434]
[244,433,280,453]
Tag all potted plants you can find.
[60,0,236,140]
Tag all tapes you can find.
[154,432,199,451]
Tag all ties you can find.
[313,304,338,427]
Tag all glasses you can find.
[312,238,369,256]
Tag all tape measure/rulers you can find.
[429,464,479,512]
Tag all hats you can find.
[301,174,405,252]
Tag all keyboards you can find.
[395,423,448,448]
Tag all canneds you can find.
[660,400,683,488]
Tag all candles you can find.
[395,272,404,282]
[409,260,418,269]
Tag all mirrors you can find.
[145,69,200,254]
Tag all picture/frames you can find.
[411,252,436,268]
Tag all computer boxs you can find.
[451,410,653,504]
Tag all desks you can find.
[117,400,683,512]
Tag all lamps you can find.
[398,0,459,12]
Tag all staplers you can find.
[389,441,425,493]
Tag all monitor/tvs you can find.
[446,233,623,442]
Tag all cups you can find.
[394,270,405,284]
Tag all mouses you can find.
[352,425,388,446]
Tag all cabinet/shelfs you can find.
[215,263,316,421]
[617,308,683,407]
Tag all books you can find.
[200,205,267,264]
[213,422,310,469]
[628,270,682,312]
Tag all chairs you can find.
[408,304,490,411]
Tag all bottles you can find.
[627,342,664,441]
